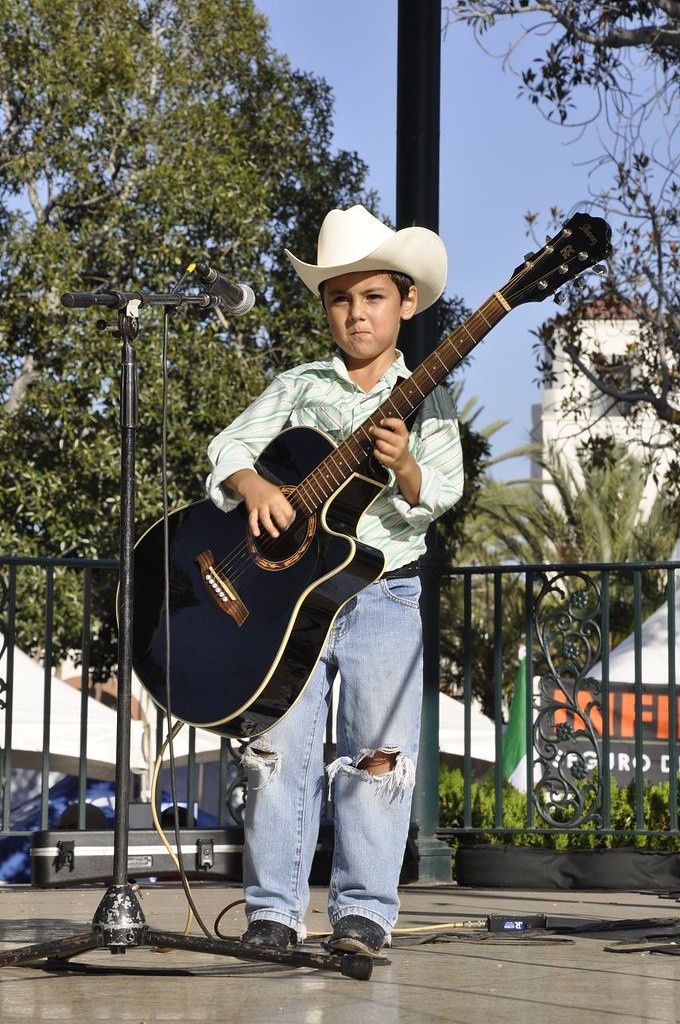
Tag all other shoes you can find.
[328,915,386,956]
[241,919,298,949]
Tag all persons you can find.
[205,204,464,956]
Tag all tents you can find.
[0,630,497,784]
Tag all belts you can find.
[381,557,421,578]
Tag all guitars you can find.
[112,212,614,743]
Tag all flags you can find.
[502,653,527,795]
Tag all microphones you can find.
[193,263,255,315]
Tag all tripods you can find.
[0,290,374,981]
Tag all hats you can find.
[285,205,448,314]
